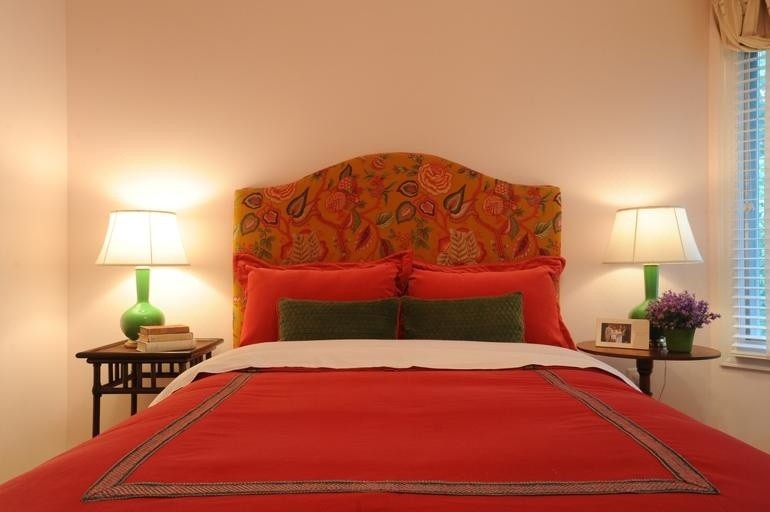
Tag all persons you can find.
[604,324,622,343]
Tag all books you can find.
[136,340,196,353]
[139,325,189,335]
[137,332,194,342]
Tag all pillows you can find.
[233,253,413,299]
[411,254,567,278]
[278,298,400,340]
[405,264,577,348]
[239,263,403,346]
[400,290,526,341]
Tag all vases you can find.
[665,328,697,352]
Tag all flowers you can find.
[643,288,722,331]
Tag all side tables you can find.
[74,337,225,438]
[575,340,722,397]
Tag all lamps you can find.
[602,207,704,342]
[94,209,194,349]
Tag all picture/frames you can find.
[595,316,651,351]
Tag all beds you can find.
[0,152,770,512]
